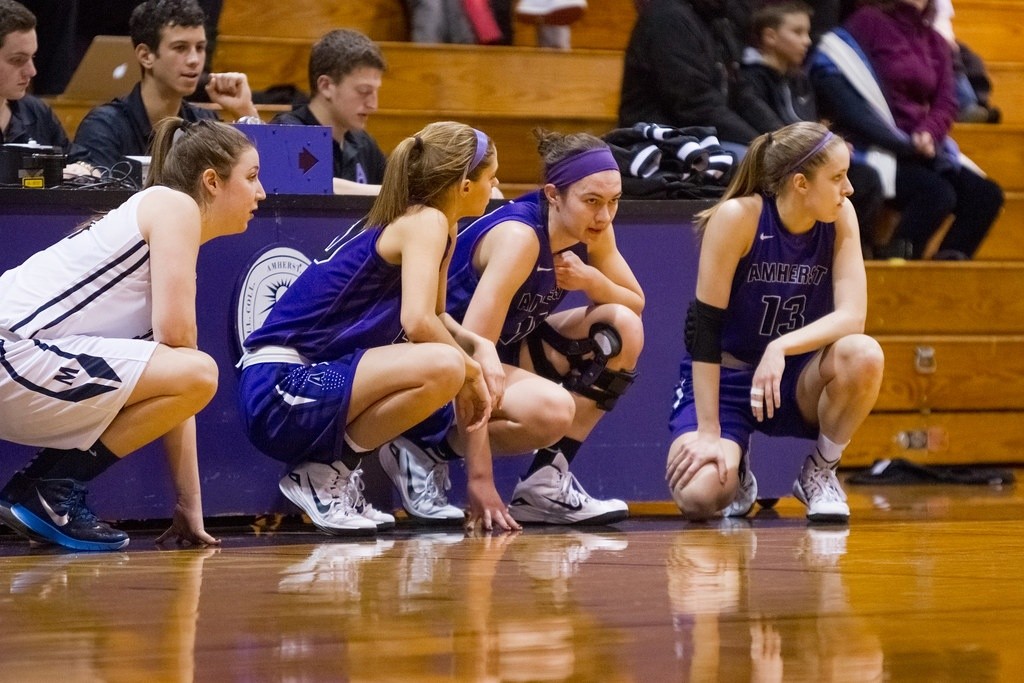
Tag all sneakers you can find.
[506,452,629,526]
[331,458,396,530]
[519,526,628,580]
[279,462,378,536]
[792,455,850,519]
[808,522,850,558]
[281,539,394,593]
[402,531,465,595]
[377,435,466,524]
[720,433,758,518]
[0,469,130,551]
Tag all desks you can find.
[0,188,819,538]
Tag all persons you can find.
[11,527,884,683]
[18,0,225,101]
[665,121,884,519]
[379,125,645,525]
[618,0,1004,259]
[74,0,260,182]
[401,0,589,49]
[0,1,72,158]
[0,117,265,550]
[267,30,388,184]
[237,122,507,534]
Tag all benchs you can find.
[42,0,1024,486]
